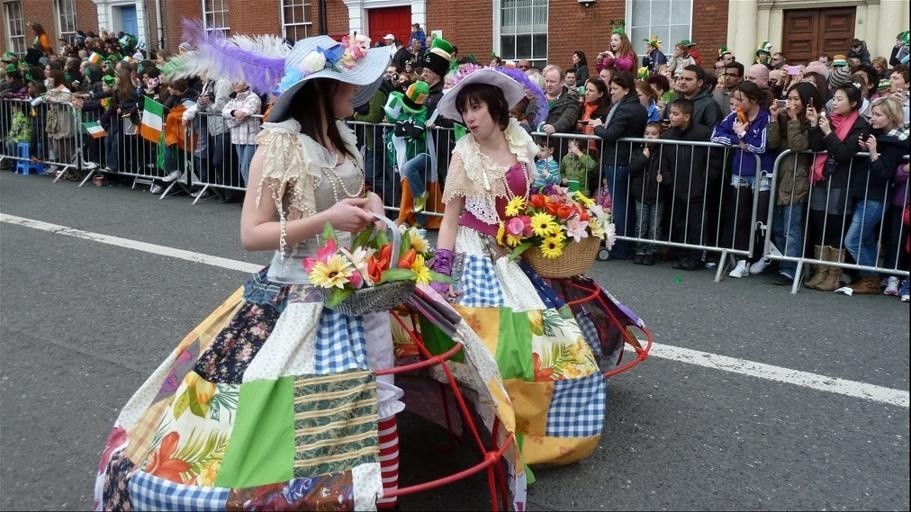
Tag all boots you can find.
[803,245,910,303]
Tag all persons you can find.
[430,62,654,470]
[528,32,910,301]
[342,23,503,232]
[95,21,529,512]
[1,19,283,202]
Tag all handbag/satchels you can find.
[44,109,59,133]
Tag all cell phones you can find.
[787,66,800,75]
[775,100,788,109]
[581,122,589,125]
[861,128,871,142]
[808,96,813,111]
[735,117,738,123]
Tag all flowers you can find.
[141,372,242,487]
[497,184,614,263]
[443,64,493,89]
[528,305,599,382]
[302,224,430,309]
[279,30,371,93]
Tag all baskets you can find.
[515,192,603,279]
[313,214,416,318]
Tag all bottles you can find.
[737,108,748,124]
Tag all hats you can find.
[643,35,665,50]
[681,39,698,48]
[717,46,732,62]
[161,16,393,122]
[436,63,550,128]
[118,35,132,48]
[419,38,455,76]
[396,80,429,114]
[0,51,31,78]
[83,51,104,66]
[829,54,852,88]
[755,41,772,57]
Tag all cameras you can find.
[152,77,159,85]
[900,90,909,98]
[208,92,215,101]
[601,54,608,59]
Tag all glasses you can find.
[721,72,739,78]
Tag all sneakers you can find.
[42,165,85,181]
[221,196,239,204]
[410,190,429,214]
[162,169,182,183]
[83,160,113,173]
[190,190,210,198]
[152,185,166,193]
[606,247,717,270]
[728,254,793,286]
[0,159,16,172]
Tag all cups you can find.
[418,229,428,239]
[96,177,104,187]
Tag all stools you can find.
[15,143,42,175]
